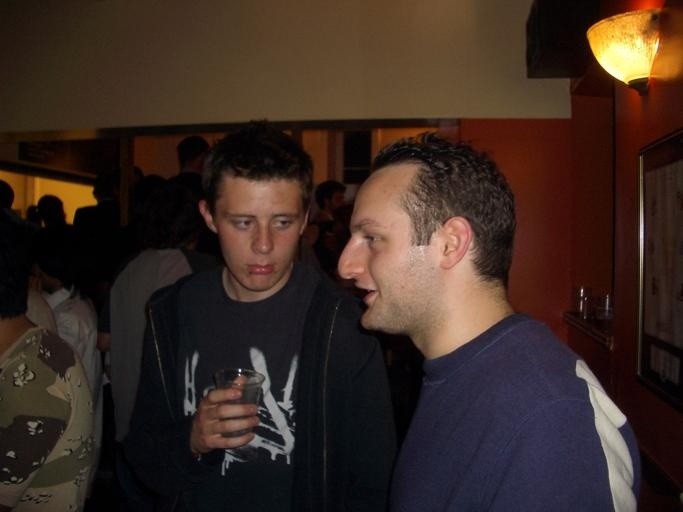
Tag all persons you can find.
[0,136,210,288]
[304,177,363,295]
[0,220,94,511]
[335,134,644,512]
[125,120,399,511]
[26,245,103,466]
[110,189,214,442]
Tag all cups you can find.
[598,288,614,313]
[572,288,593,315]
[214,367,266,438]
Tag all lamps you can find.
[585,7,660,96]
